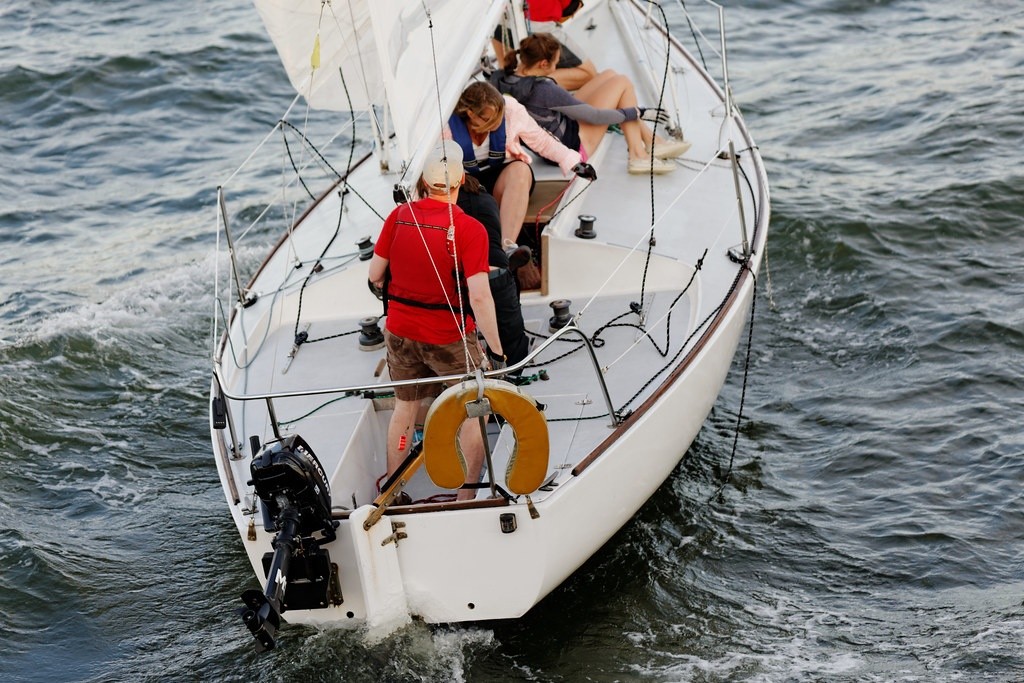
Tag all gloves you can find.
[572,162,597,181]
[636,106,670,125]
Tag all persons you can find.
[486,24,692,176]
[436,79,597,269]
[368,139,507,501]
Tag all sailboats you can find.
[208,0,772,651]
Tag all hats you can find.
[423,140,463,190]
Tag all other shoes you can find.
[650,139,690,158]
[387,491,411,508]
[627,154,674,174]
[503,239,531,272]
